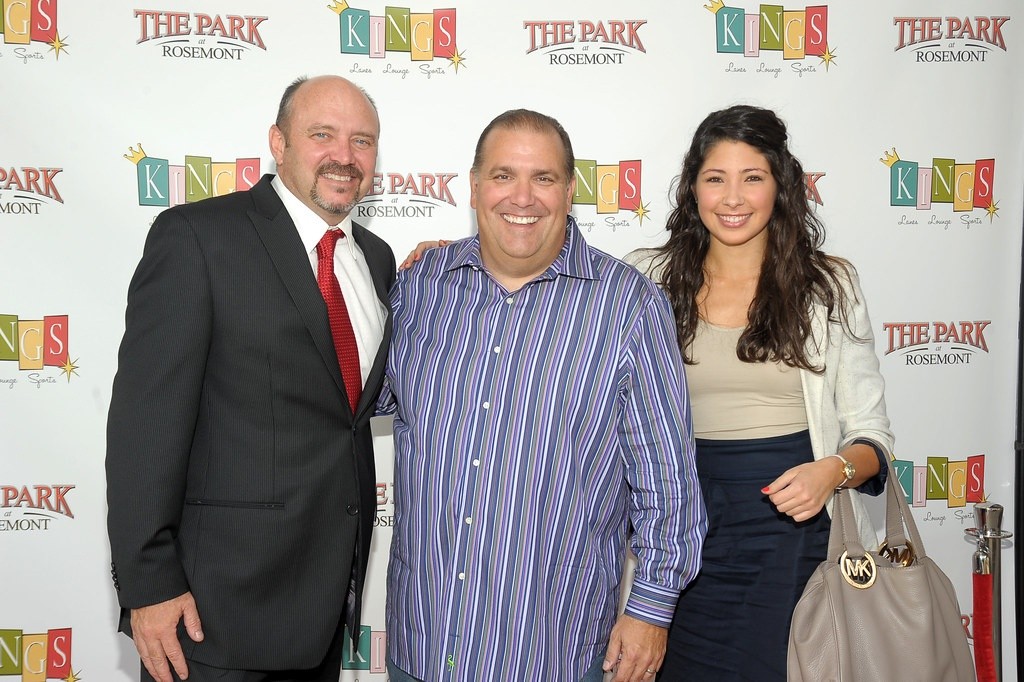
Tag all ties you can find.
[315,230,361,416]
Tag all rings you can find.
[647,669,655,674]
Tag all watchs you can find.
[831,455,855,489]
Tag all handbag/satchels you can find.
[786,437,976,682]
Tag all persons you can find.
[107,75,397,682]
[372,110,709,682]
[399,105,895,682]
